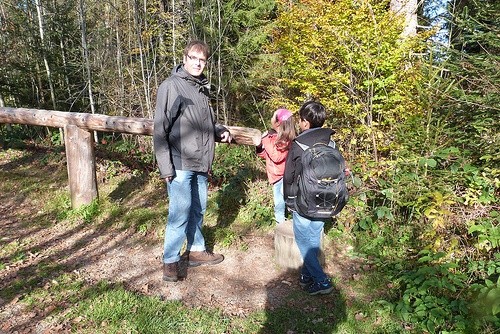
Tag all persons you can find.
[257,109,300,226]
[152,41,224,283]
[284,101,339,297]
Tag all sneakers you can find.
[299,274,313,286]
[163,263,178,282]
[182,250,224,267]
[306,279,333,296]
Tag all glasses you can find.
[187,54,207,65]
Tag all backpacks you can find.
[295,138,347,219]
